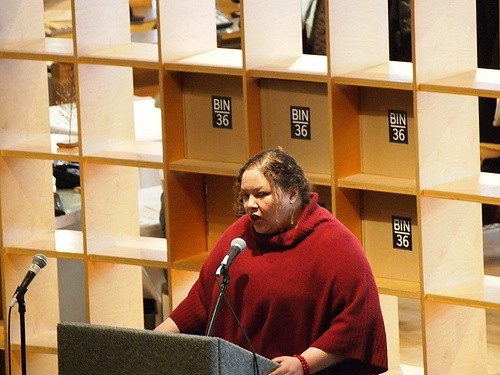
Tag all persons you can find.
[153,149,389,375]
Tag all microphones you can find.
[9,254,47,309]
[215,237,247,277]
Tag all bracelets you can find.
[293,354,311,375]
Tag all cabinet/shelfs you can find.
[0,0,500,375]
